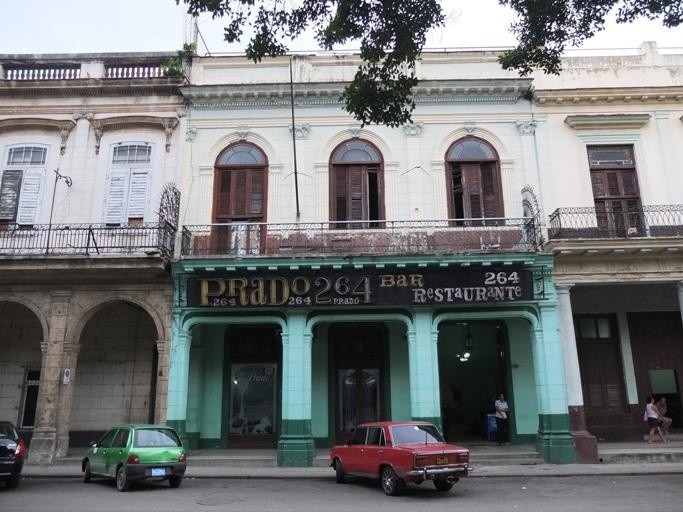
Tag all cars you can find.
[0,420,26,489]
[327,421,471,497]
[82,423,187,492]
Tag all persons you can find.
[643,395,667,444]
[494,392,510,443]
[657,397,672,435]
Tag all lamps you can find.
[462,323,474,352]
